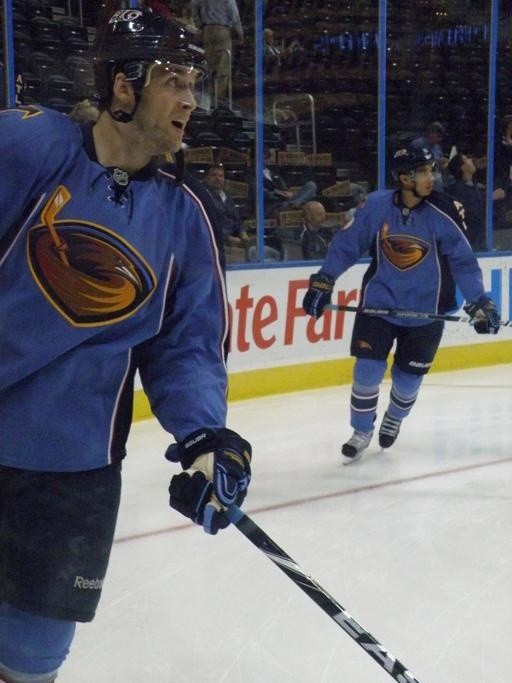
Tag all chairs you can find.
[0,0,511,264]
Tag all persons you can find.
[0,0,512,264]
[301,138,500,457]
[1,7,255,682]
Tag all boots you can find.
[379,410,401,447]
[342,413,376,458]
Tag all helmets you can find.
[386,143,437,181]
[92,7,212,97]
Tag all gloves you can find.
[463,293,501,335]
[303,273,335,320]
[164,428,252,536]
